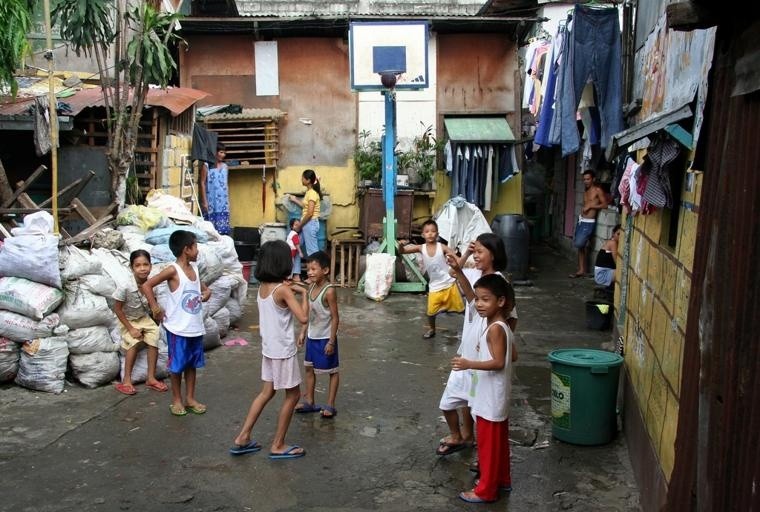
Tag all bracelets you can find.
[328,342,335,346]
[153,308,161,313]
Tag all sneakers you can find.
[423,329,436,338]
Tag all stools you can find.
[593,284,608,298]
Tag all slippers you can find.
[458,489,485,502]
[568,272,585,279]
[268,444,304,459]
[145,381,169,392]
[321,405,336,418]
[295,402,321,414]
[473,478,513,491]
[169,403,188,416]
[115,383,136,395]
[435,441,466,455]
[229,440,262,455]
[185,403,207,415]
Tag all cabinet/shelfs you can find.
[358,188,415,244]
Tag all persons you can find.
[569,170,608,278]
[228,240,309,459]
[286,219,305,283]
[419,246,466,295]
[294,250,340,418]
[453,275,519,503]
[201,142,231,236]
[436,232,518,457]
[594,224,620,287]
[289,170,322,257]
[112,250,168,394]
[400,220,466,340]
[143,231,207,415]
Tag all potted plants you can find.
[354,129,382,186]
[396,152,413,187]
[413,120,444,191]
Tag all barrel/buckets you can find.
[241,262,252,283]
[584,299,613,331]
[490,213,531,281]
[259,222,287,248]
[286,197,327,259]
